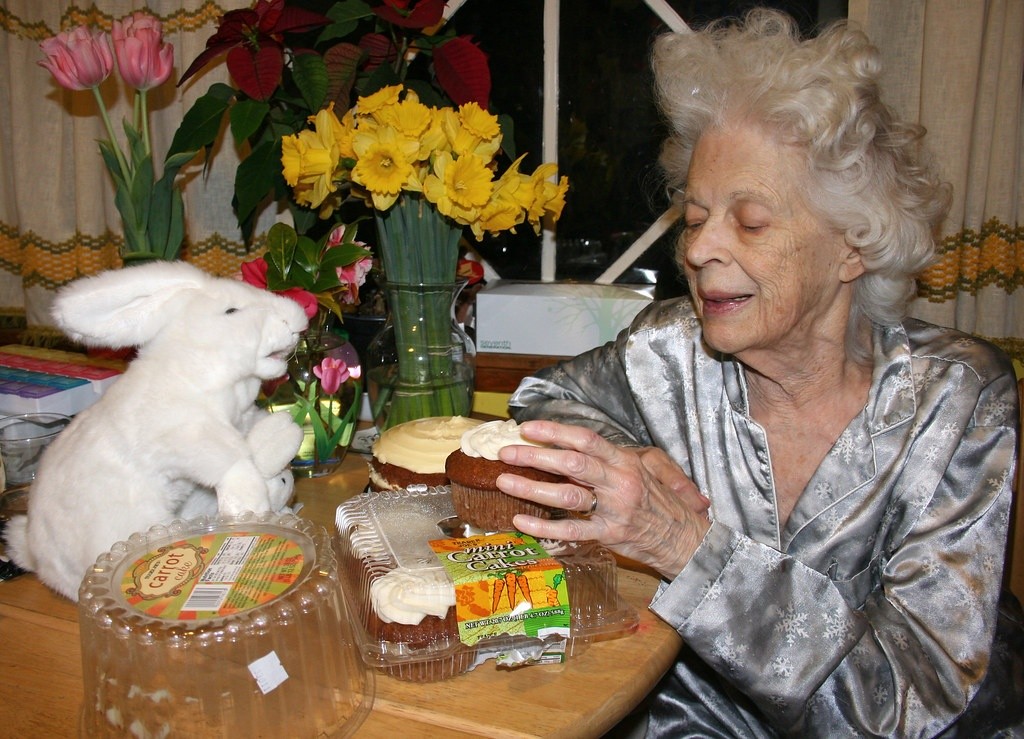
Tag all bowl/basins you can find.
[73,512,376,739]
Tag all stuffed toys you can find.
[6,261,312,604]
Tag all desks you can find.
[0,410,694,739]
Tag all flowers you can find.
[259,356,365,466]
[35,0,602,340]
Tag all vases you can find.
[379,275,480,434]
[254,324,363,478]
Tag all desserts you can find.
[336,498,500,683]
[444,417,561,532]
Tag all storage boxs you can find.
[477,277,657,357]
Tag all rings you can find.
[578,488,598,518]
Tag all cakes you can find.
[367,414,495,496]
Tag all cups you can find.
[0,412,71,490]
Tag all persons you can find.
[497,9,1020,738]
